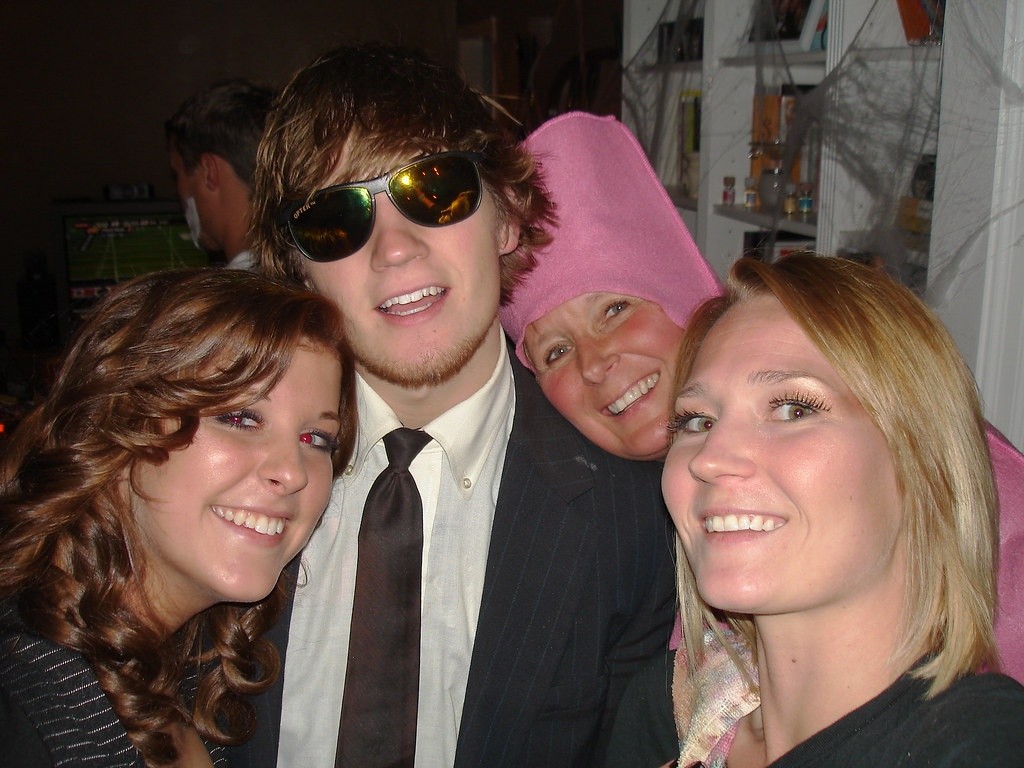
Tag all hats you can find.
[497,108,728,374]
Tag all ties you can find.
[335,428,433,768]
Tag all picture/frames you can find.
[738,0,824,56]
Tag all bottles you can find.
[743,178,757,207]
[783,183,796,214]
[723,176,736,206]
[799,183,813,213]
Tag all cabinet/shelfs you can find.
[619,0,1024,449]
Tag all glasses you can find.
[278,149,490,263]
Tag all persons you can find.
[496,110,1024,767]
[220,38,678,768]
[608,246,1024,767]
[163,78,282,270]
[0,268,356,768]
